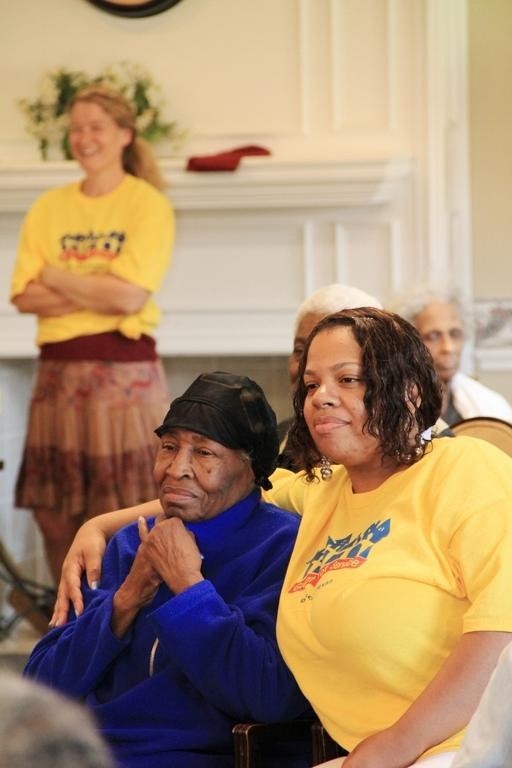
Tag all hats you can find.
[154,370,279,491]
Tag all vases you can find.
[44,142,67,161]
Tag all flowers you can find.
[14,59,193,151]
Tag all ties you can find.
[441,390,463,426]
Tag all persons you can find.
[8,86,177,606]
[1,282,511,768]
